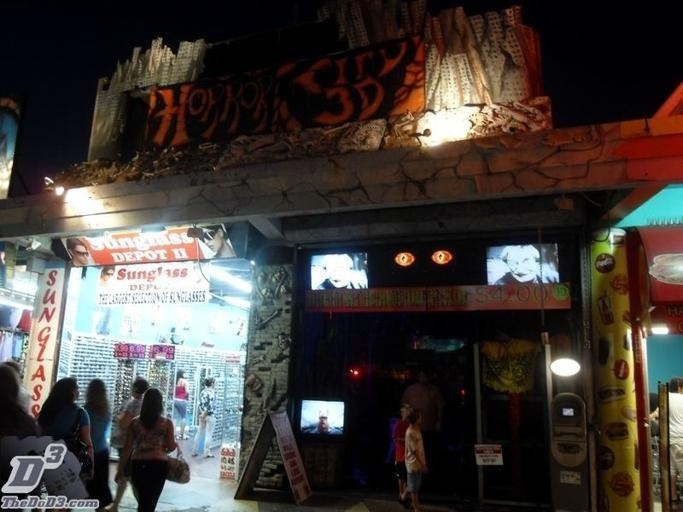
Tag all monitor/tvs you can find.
[481,238,565,286]
[305,247,374,291]
[297,397,349,437]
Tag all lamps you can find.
[41,174,65,197]
[13,236,42,253]
[405,125,432,147]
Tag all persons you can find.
[188,226,236,259]
[67,237,88,267]
[493,245,559,285]
[649,378,683,502]
[313,253,368,290]
[310,410,343,435]
[390,372,443,512]
[1,362,215,512]
[101,265,114,283]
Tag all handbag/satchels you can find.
[75,440,92,476]
[167,457,190,485]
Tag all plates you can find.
[647,253,682,285]
